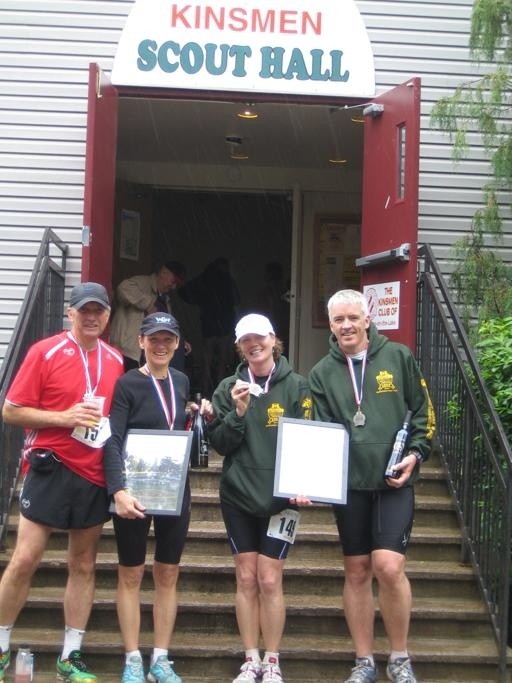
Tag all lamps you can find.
[226,99,259,159]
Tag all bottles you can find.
[13,643,33,683]
[191,394,207,471]
[384,409,413,480]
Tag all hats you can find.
[140,311,180,338]
[233,313,275,344]
[69,281,110,311]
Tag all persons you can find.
[189,256,240,391]
[208,312,312,683]
[104,312,216,683]
[264,262,285,299]
[0,280,130,682]
[312,290,436,683]
[111,259,192,373]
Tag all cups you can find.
[84,395,107,425]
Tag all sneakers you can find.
[147,655,182,682]
[261,654,282,682]
[385,654,417,682]
[121,655,145,682]
[231,656,263,682]
[56,650,99,682]
[345,657,379,682]
[0,646,10,679]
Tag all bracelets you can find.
[408,450,424,465]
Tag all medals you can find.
[353,405,366,426]
[238,381,263,397]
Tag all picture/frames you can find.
[108,427,194,518]
[272,415,350,506]
